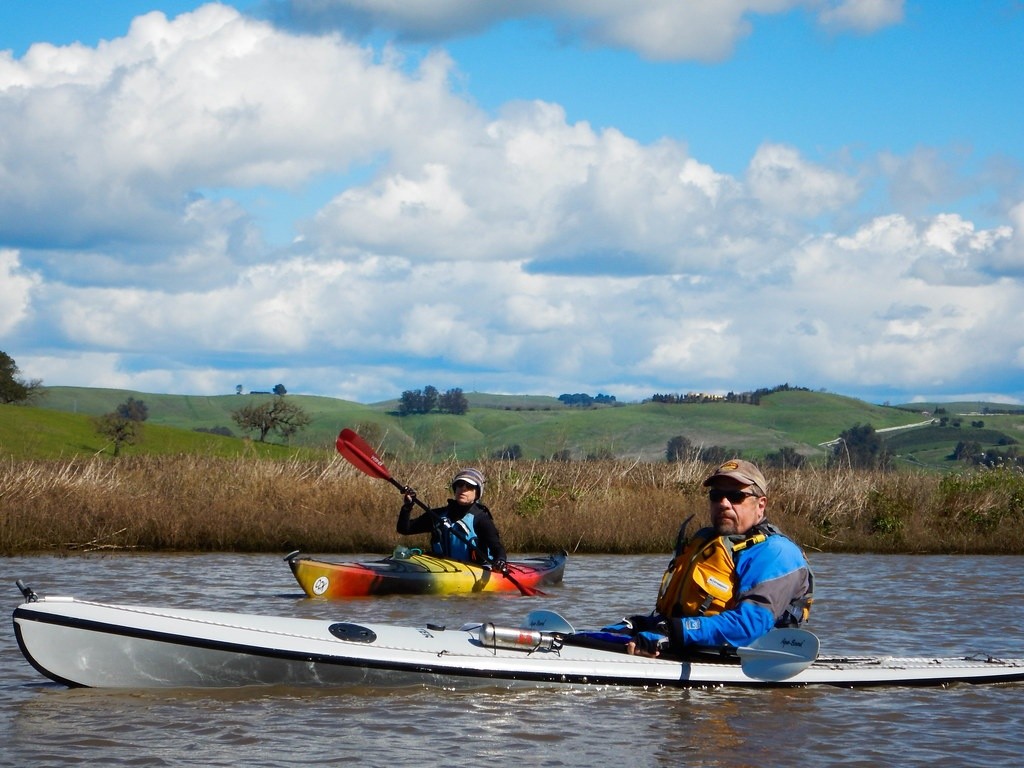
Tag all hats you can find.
[703,459,767,496]
[452,468,484,502]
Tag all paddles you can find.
[333,425,534,597]
[522,607,825,685]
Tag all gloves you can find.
[493,559,508,575]
[401,485,417,510]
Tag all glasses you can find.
[710,489,760,504]
[456,481,477,491]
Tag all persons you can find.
[396,468,508,574]
[600,459,814,658]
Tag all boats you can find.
[10,572,1024,688]
[283,547,571,600]
